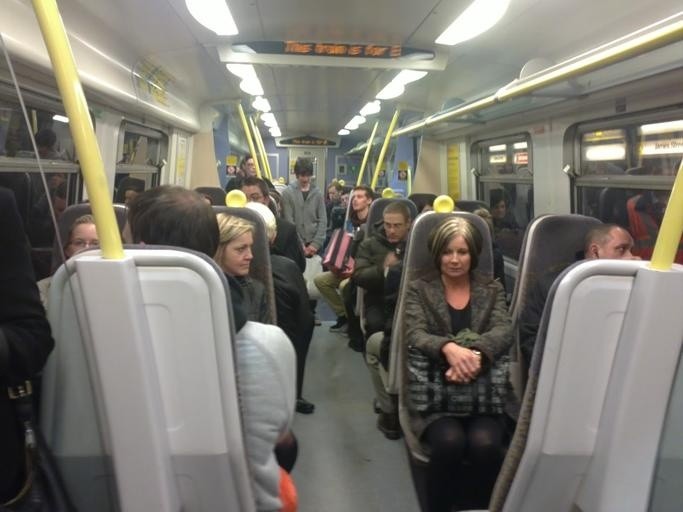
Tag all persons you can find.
[215,213,299,474]
[240,177,315,415]
[41,184,296,512]
[400,216,515,512]
[521,221,641,369]
[319,181,419,440]
[615,158,682,267]
[21,129,63,223]
[490,193,522,260]
[38,214,99,309]
[223,155,275,196]
[282,157,327,311]
[0,186,54,512]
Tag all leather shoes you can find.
[296,398,315,413]
[374,395,400,439]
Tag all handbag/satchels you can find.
[405,346,517,415]
[322,227,357,279]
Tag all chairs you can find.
[326,178,496,417]
[508,211,605,410]
[490,258,683,511]
[378,192,512,465]
[37,243,259,512]
[51,179,289,326]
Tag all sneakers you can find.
[330,316,347,330]
